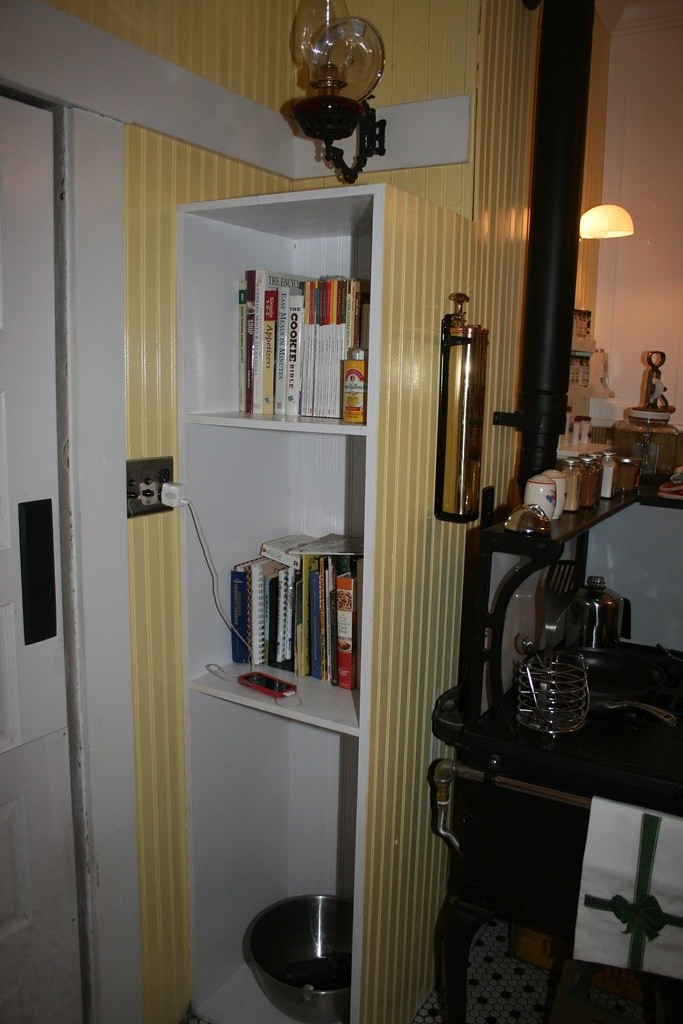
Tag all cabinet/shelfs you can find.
[174,186,469,1024]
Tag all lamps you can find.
[294,0,387,185]
[579,204,634,241]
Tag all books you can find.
[239,270,368,418]
[229,533,362,691]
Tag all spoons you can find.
[514,633,577,722]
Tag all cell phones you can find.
[237,671,297,698]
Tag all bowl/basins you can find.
[242,893,352,1024]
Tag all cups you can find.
[524,473,566,520]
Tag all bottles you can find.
[342,347,367,423]
[564,408,679,513]
[566,576,627,686]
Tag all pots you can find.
[544,646,679,727]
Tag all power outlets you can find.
[126,456,174,518]
